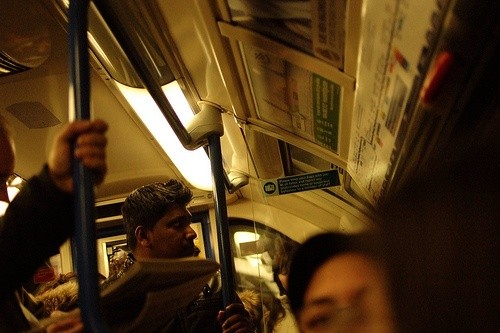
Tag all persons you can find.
[31,250,128,315]
[261,238,303,333]
[98,177,257,333]
[0,119,111,333]
[284,226,401,333]
[1,110,17,207]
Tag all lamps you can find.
[234,231,264,264]
[112,80,218,193]
